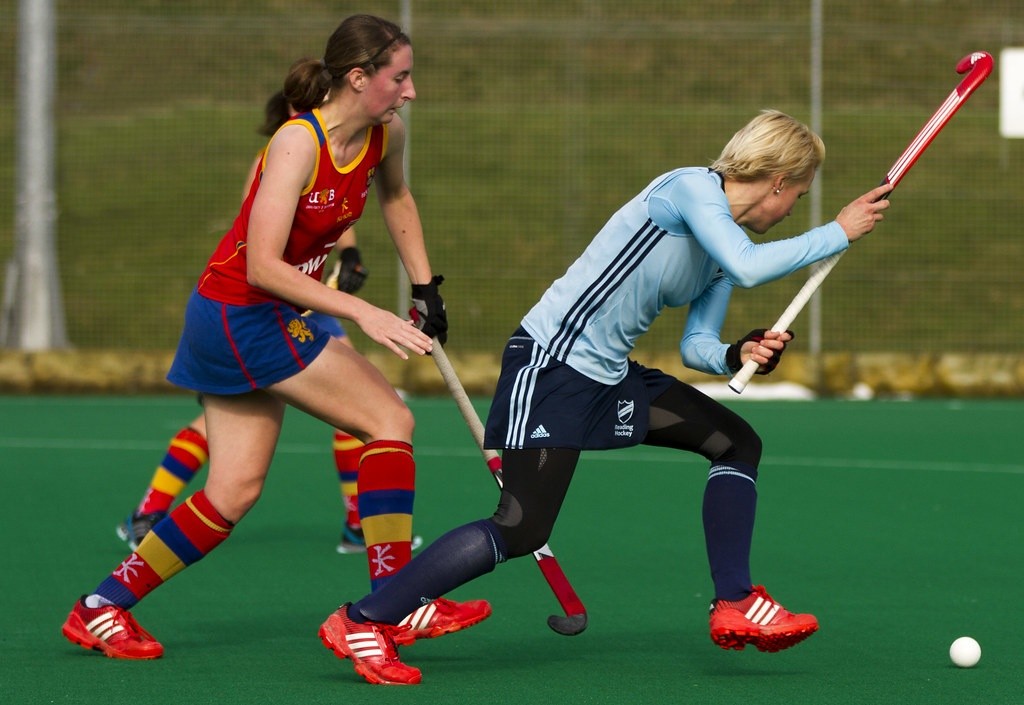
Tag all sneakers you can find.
[389,596,493,647]
[61,595,164,659]
[319,603,422,685]
[117,506,166,551]
[336,519,422,555]
[709,585,819,652]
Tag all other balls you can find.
[852,381,873,401]
[949,637,982,668]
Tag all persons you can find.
[317,108,893,687]
[60,13,492,661]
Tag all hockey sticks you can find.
[727,51,995,396]
[408,305,588,637]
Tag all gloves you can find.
[337,247,369,295]
[405,276,451,356]
